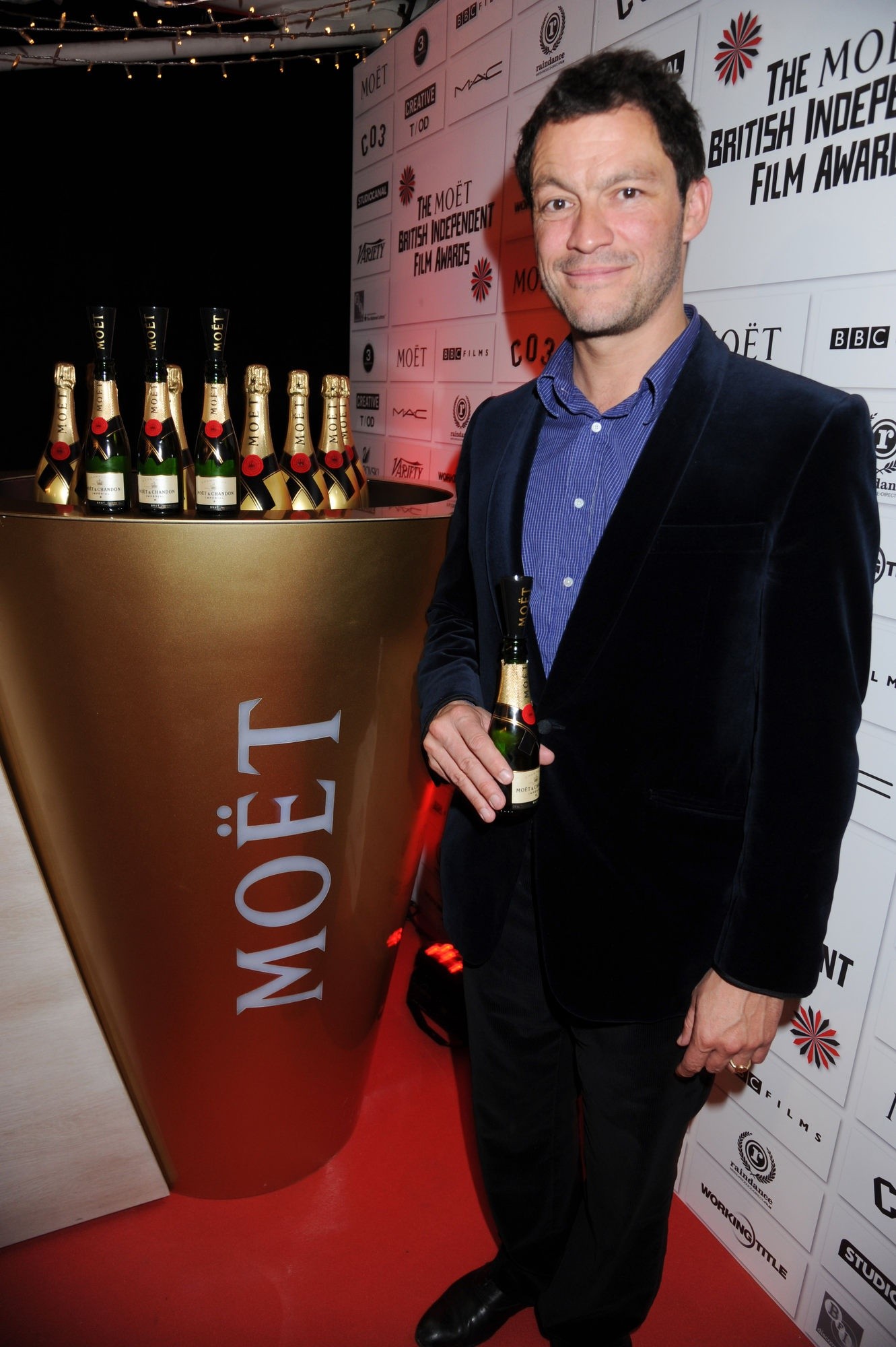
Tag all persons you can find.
[413,46,877,1347]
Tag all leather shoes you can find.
[415,1263,527,1347]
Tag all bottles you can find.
[280,371,331,510]
[37,306,132,519]
[239,364,293,510]
[487,576,541,812]
[317,374,368,510]
[138,307,240,519]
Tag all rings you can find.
[730,1058,753,1073]
